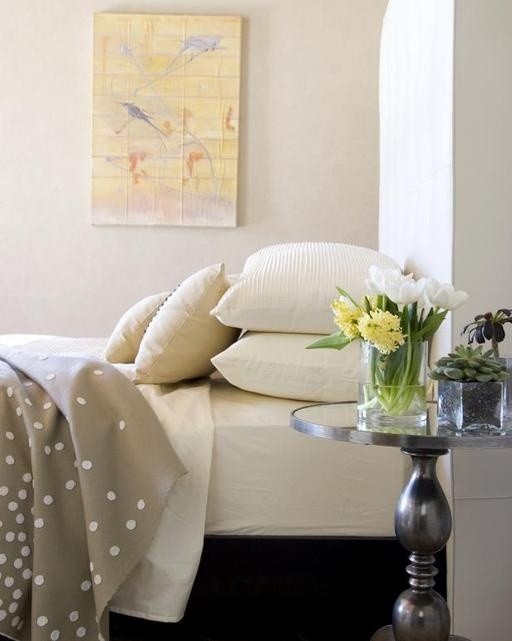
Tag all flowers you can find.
[304,266,468,414]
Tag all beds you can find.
[0,335,413,540]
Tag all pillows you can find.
[133,262,242,385]
[208,241,400,336]
[105,291,172,363]
[209,331,430,400]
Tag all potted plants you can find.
[462,308,512,422]
[427,308,502,432]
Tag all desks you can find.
[290,401,512,641]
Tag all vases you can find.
[357,342,427,423]
